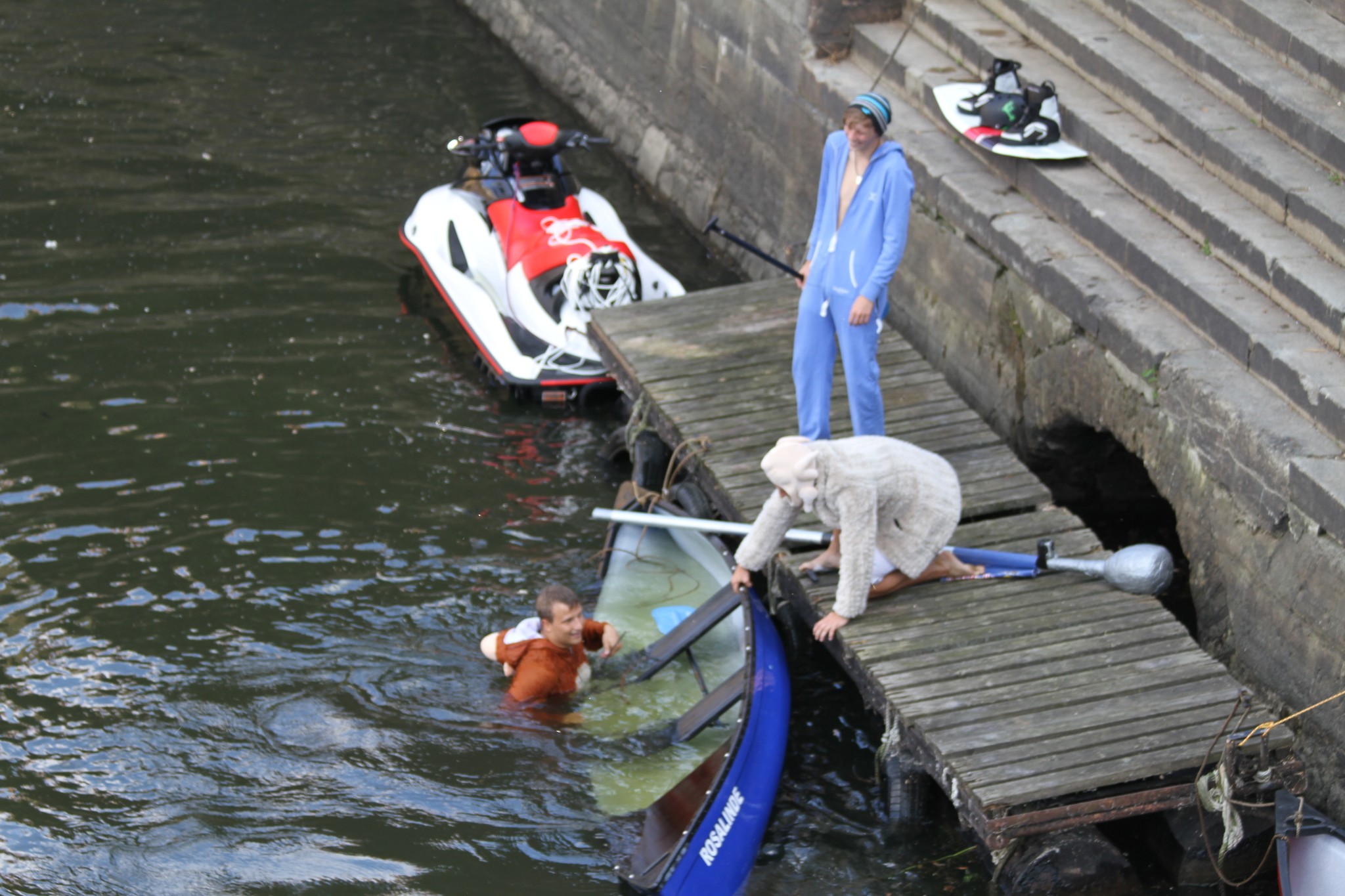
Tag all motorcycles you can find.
[400,115,688,414]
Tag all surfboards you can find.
[931,59,1095,163]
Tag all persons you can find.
[479,585,623,702]
[730,434,985,641]
[792,93,915,440]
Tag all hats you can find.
[848,92,892,137]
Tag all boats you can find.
[562,478,794,896]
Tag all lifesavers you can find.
[868,740,931,826]
[601,422,674,495]
[996,828,1139,892]
[664,480,714,521]
